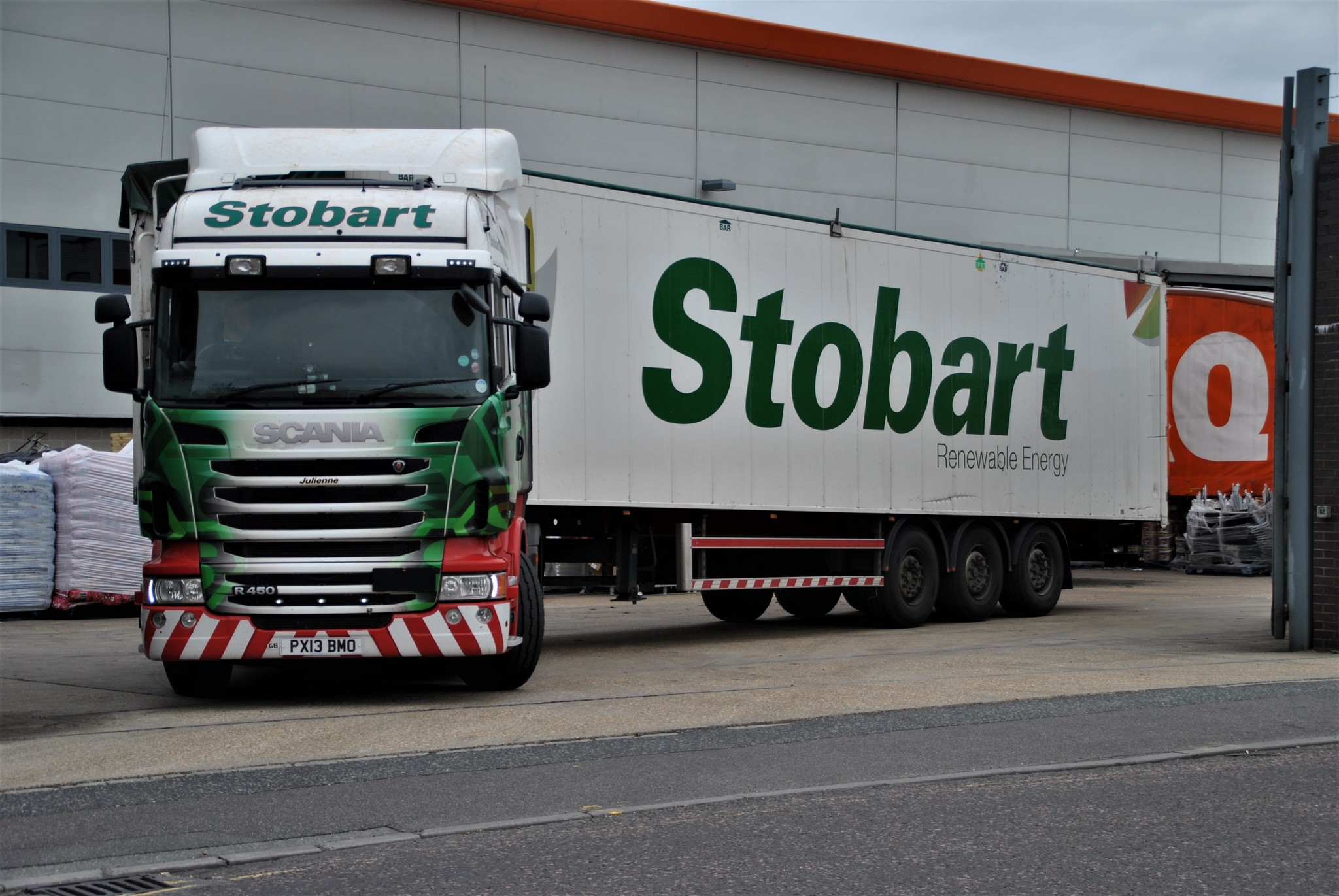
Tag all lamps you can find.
[702,179,735,192]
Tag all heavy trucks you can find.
[93,127,1167,698]
